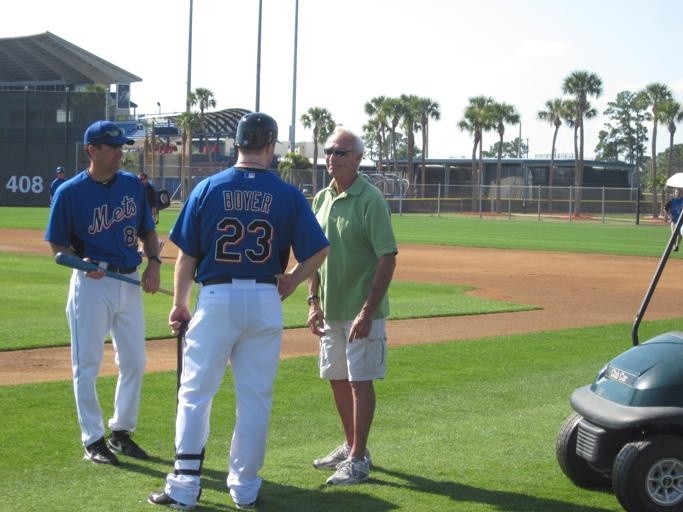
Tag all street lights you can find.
[157,101,163,114]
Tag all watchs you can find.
[150,255,162,264]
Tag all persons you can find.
[45,120,161,464]
[307,130,398,486]
[138,173,164,256]
[147,112,331,512]
[50,167,69,208]
[663,189,683,251]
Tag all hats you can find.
[83,120,135,146]
[234,112,282,148]
[56,167,64,173]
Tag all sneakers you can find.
[82,436,118,465]
[107,431,147,457]
[312,444,373,485]
[147,490,195,510]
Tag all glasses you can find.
[323,147,355,156]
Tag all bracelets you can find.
[307,294,320,306]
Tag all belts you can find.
[88,258,137,275]
[201,275,278,286]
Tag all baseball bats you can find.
[55,252,175,296]
